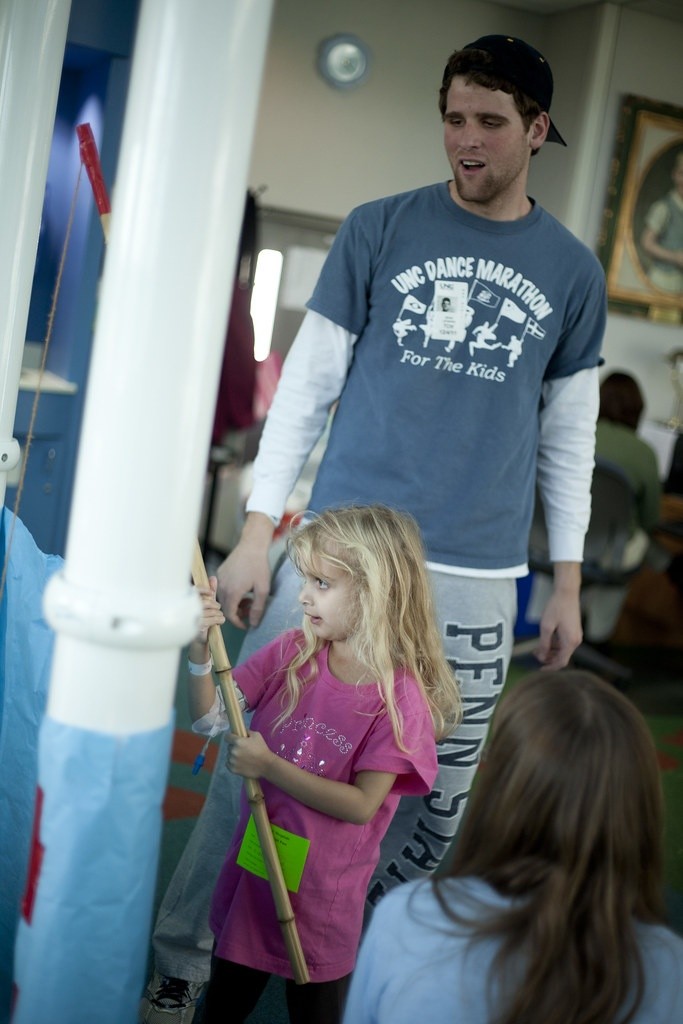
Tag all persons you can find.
[188,504,461,1024]
[340,668,683,1024]
[135,35,609,1024]
[526,367,682,666]
[640,143,683,296]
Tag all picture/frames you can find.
[591,89,683,329]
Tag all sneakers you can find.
[138,971,205,1023]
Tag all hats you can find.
[464,32,568,148]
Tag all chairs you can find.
[510,525,650,681]
